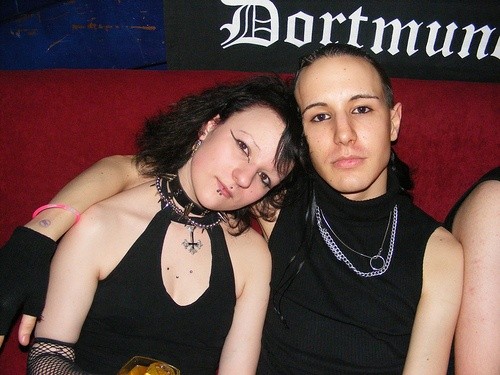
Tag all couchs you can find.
[0,68,500,375]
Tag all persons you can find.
[27,75,311,375]
[442,165,500,375]
[0,40,463,375]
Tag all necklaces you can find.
[154,174,223,254]
[315,205,399,276]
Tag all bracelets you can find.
[33,203,80,225]
